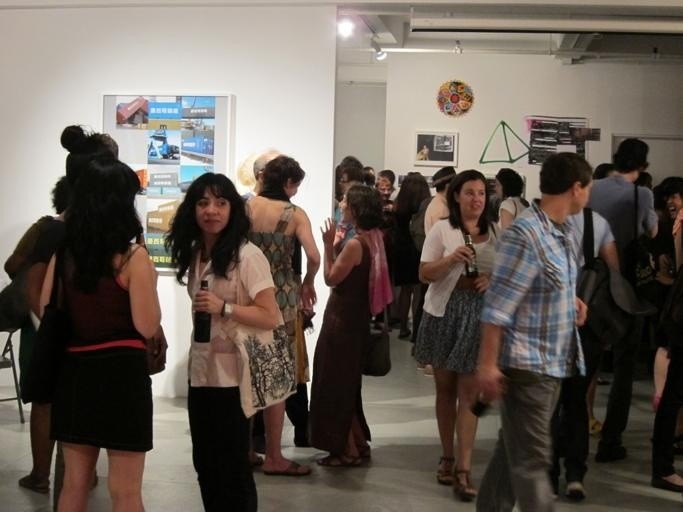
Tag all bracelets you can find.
[220,301,225,317]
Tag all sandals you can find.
[587,415,603,435]
[317,444,373,466]
[650,472,683,493]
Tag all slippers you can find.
[252,455,312,477]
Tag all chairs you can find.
[0,280,33,421]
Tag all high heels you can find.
[651,394,661,412]
[437,454,476,501]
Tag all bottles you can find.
[193,279,212,342]
[462,231,478,279]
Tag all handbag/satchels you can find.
[143,324,168,375]
[628,235,665,302]
[363,329,394,379]
[578,266,633,345]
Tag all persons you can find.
[242,155,322,478]
[306,184,384,469]
[462,152,596,511]
[37,156,163,511]
[1,175,100,495]
[234,150,313,454]
[165,171,282,511]
[58,121,122,177]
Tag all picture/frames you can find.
[412,130,459,168]
[96,89,237,277]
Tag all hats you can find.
[431,167,456,187]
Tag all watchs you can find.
[223,302,233,316]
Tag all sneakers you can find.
[397,326,435,377]
[596,444,626,463]
[18,472,52,494]
[551,459,588,501]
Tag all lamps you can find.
[359,15,387,61]
